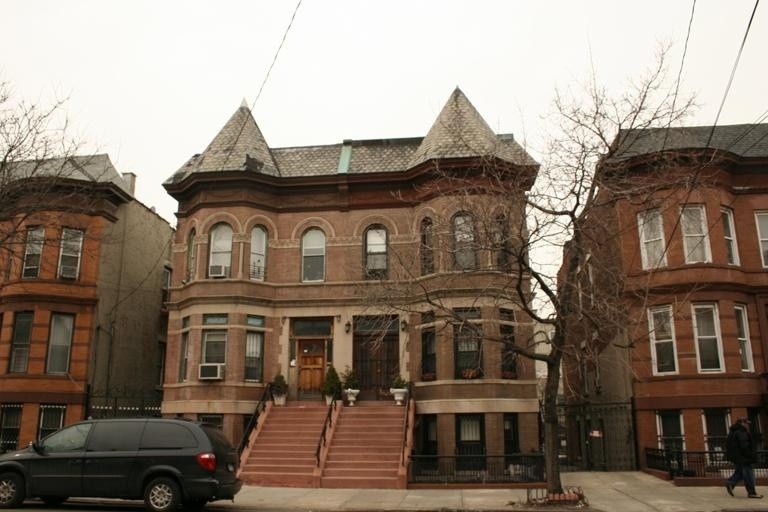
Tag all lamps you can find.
[400,318,408,332]
[345,320,350,334]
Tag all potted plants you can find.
[271,373,288,406]
[390,375,409,406]
[322,364,340,404]
[343,370,361,405]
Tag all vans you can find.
[0,415,247,512]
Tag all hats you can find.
[736,415,751,424]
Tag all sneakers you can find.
[747,494,764,498]
[725,480,734,496]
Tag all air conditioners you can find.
[198,363,224,379]
[208,264,226,277]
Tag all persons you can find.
[722,415,763,498]
